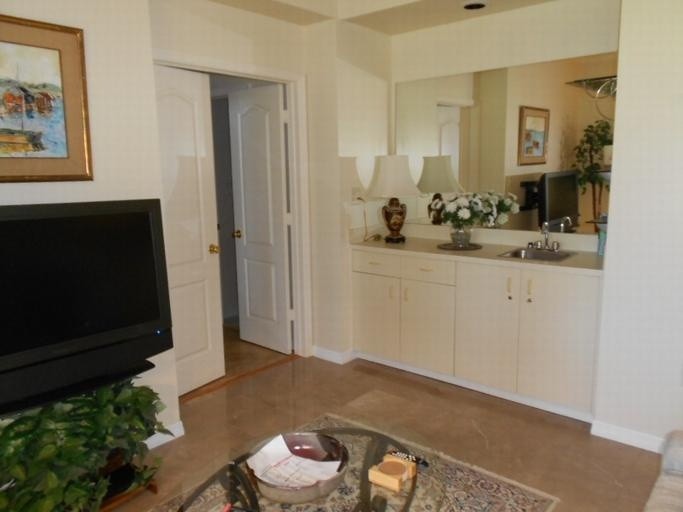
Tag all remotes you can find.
[389,450,429,470]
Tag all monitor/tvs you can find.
[520,172,581,234]
[0,199,174,424]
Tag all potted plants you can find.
[0,378,175,512]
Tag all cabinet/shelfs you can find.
[349,244,456,377]
[455,255,603,414]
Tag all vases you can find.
[439,222,482,251]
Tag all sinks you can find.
[498,246,575,263]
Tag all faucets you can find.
[541,221,551,250]
[560,216,572,232]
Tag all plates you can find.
[246,431,351,503]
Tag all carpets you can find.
[148,411,562,512]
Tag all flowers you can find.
[428,192,483,230]
[483,188,520,227]
[572,119,613,233]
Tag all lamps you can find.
[418,155,467,226]
[366,153,418,244]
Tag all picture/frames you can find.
[518,106,550,165]
[0,15,95,181]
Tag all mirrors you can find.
[393,50,618,236]
